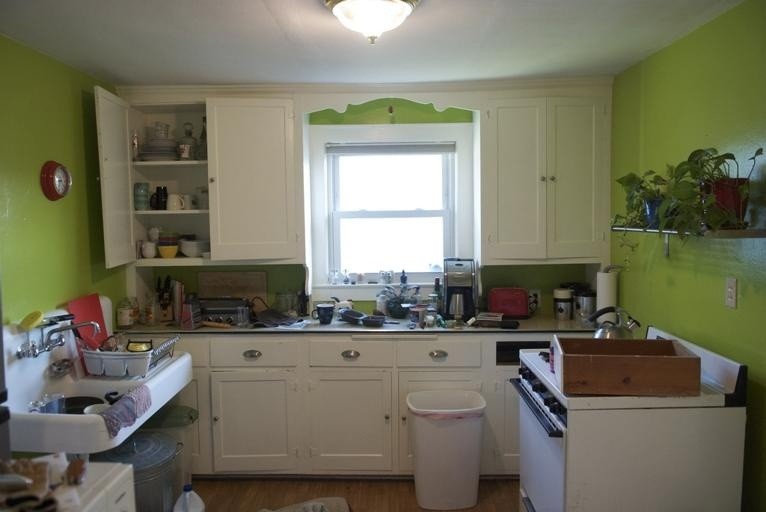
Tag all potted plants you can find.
[668,149,763,230]
[615,165,676,230]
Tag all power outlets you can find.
[724,279,738,309]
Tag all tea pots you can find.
[586,305,641,340]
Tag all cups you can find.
[44,393,64,414]
[149,186,168,209]
[134,182,148,209]
[182,194,198,210]
[26,401,46,414]
[166,194,185,210]
[311,304,334,324]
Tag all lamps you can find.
[322,0,423,45]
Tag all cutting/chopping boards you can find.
[198,271,267,312]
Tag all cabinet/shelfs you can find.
[307,333,485,473]
[488,75,613,266]
[123,333,301,475]
[72,463,135,512]
[95,86,304,271]
[496,372,550,475]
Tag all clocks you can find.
[40,160,72,202]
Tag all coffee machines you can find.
[442,258,479,325]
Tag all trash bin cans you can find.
[406,390,486,510]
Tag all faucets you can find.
[22,322,107,353]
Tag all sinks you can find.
[10,380,145,453]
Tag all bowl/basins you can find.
[338,308,365,323]
[142,242,156,258]
[155,120,170,139]
[158,244,179,258]
[363,316,386,327]
[82,403,112,414]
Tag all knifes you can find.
[156,274,174,310]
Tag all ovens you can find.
[510,349,748,512]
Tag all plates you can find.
[138,138,179,162]
[179,238,210,256]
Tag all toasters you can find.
[487,288,529,318]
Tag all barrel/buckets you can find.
[171,484,205,512]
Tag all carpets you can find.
[272,496,350,511]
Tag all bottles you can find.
[132,297,140,324]
[179,122,198,161]
[434,314,447,327]
[432,277,442,311]
[145,296,160,327]
[427,293,438,311]
[116,296,134,329]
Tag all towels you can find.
[123,383,153,421]
[98,394,137,440]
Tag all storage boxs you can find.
[553,333,701,398]
